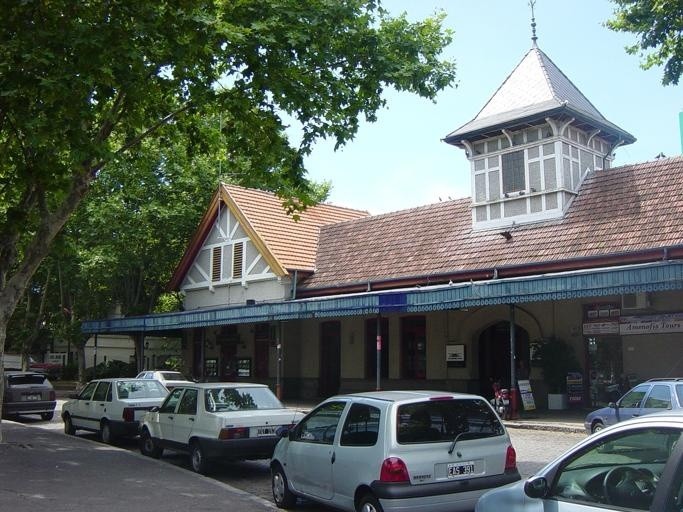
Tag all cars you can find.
[3,371,57,421]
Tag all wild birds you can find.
[518,190,525,196]
[529,186,536,193]
[655,153,661,160]
[504,192,511,199]
[661,152,667,158]
